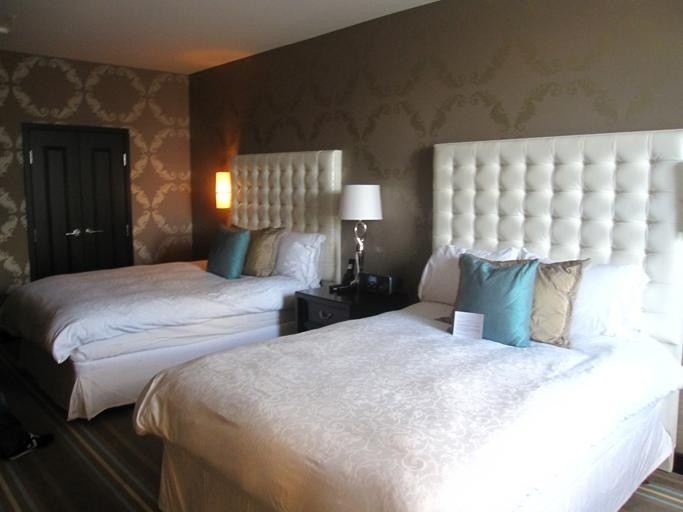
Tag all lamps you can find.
[335,184,383,294]
[214,170,232,209]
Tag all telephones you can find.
[329,258,357,296]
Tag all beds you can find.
[1,148,344,423]
[132,126,682,510]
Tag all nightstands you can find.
[296,284,406,332]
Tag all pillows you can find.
[446,256,539,348]
[229,224,285,278]
[272,232,325,284]
[205,223,250,280]
[531,258,595,349]
[417,242,525,309]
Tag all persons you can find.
[0,396,57,461]
